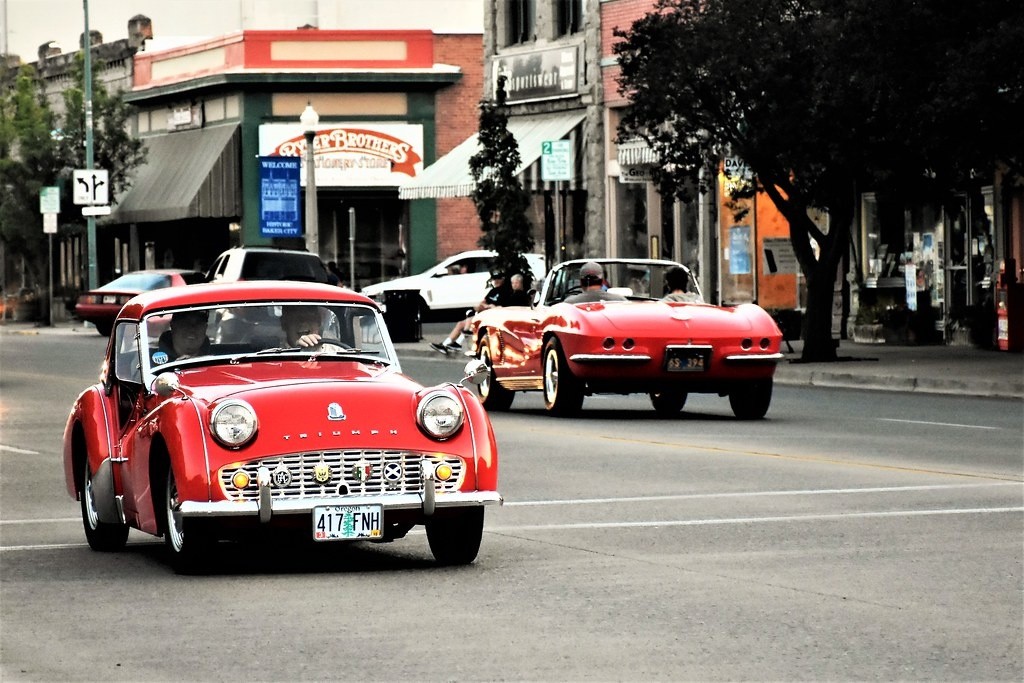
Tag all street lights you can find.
[300,105,319,253]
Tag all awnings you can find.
[95,122,241,225]
[396,108,585,198]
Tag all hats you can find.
[580,261,603,281]
[491,272,502,280]
[173,309,209,323]
[281,305,318,316]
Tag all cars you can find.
[464,258,785,421]
[62,280,503,575]
[74,269,205,340]
[359,249,546,322]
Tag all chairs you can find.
[157,331,211,365]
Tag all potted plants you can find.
[882,307,922,345]
[853,305,888,345]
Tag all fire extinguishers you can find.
[997,301,1008,350]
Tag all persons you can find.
[461,272,530,336]
[280,306,349,355]
[149,310,214,365]
[660,267,707,304]
[564,263,629,303]
[430,270,502,354]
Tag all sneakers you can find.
[430,342,448,355]
[447,341,462,350]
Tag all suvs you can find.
[206,244,330,290]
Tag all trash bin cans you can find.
[384,290,422,343]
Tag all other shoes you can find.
[461,329,474,336]
